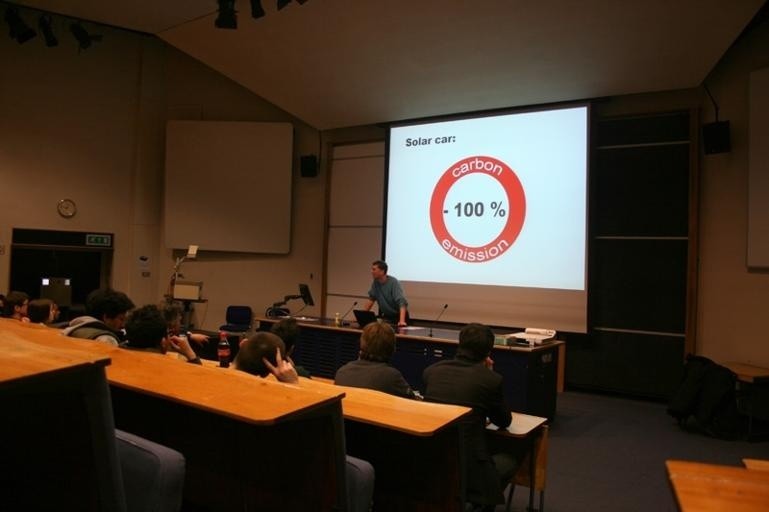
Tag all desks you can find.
[662,457,769,511]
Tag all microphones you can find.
[428,304,449,336]
[339,302,357,325]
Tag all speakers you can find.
[701,122,732,154]
[300,155,319,177]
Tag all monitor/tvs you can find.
[300,283,315,306]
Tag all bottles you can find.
[218,332,231,368]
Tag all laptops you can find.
[354,309,378,326]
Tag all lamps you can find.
[210,0,311,31]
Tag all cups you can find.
[336,313,343,327]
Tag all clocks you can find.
[56,197,78,218]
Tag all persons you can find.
[349,259,411,328]
[60,286,135,345]
[0,290,69,329]
[158,295,213,356]
[334,318,419,404]
[121,304,204,365]
[227,332,301,384]
[419,321,530,504]
